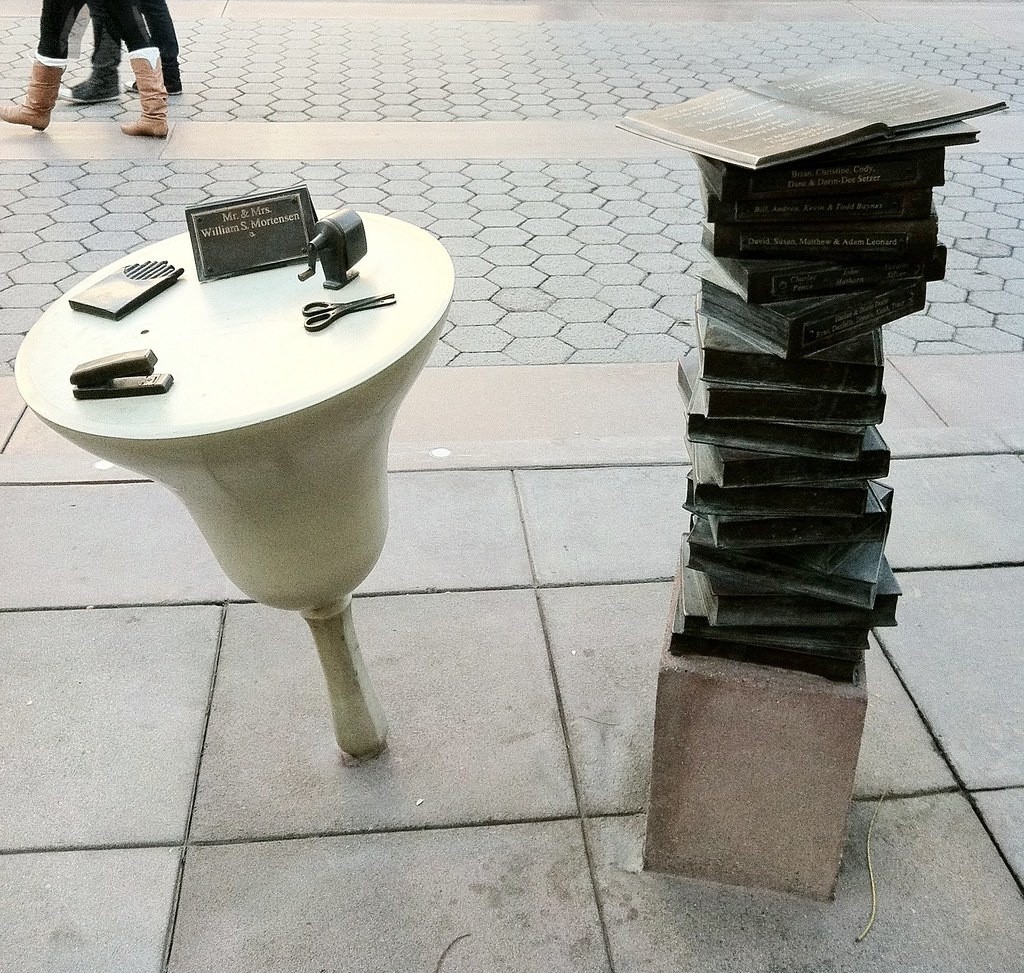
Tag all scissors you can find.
[301,293,397,332]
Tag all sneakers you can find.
[125,80,183,96]
[58,76,121,103]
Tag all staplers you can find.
[68,348,174,401]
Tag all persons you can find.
[0,0,183,139]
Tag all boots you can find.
[121,46,169,139]
[0,49,68,130]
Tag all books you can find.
[614,60,1011,685]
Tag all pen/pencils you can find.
[120,260,185,280]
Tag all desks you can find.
[13,211,456,761]
